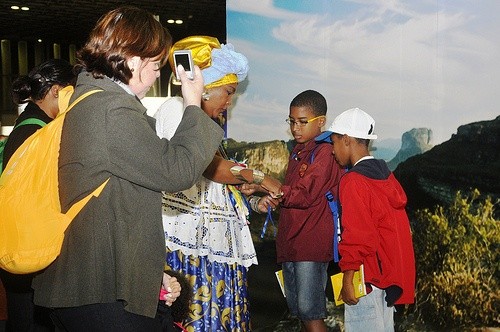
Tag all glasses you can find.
[286,117,325,127]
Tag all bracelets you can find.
[246,195,261,214]
[229,165,265,185]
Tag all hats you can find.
[314,107,378,142]
[168,35,249,90]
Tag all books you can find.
[330,264,367,306]
[275,269,286,297]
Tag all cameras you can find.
[172,49,194,82]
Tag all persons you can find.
[152,35,284,332]
[238,89,346,332]
[315,107,416,332]
[2,59,78,172]
[31,7,204,332]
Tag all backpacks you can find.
[0,86,110,274]
[0,118,47,175]
